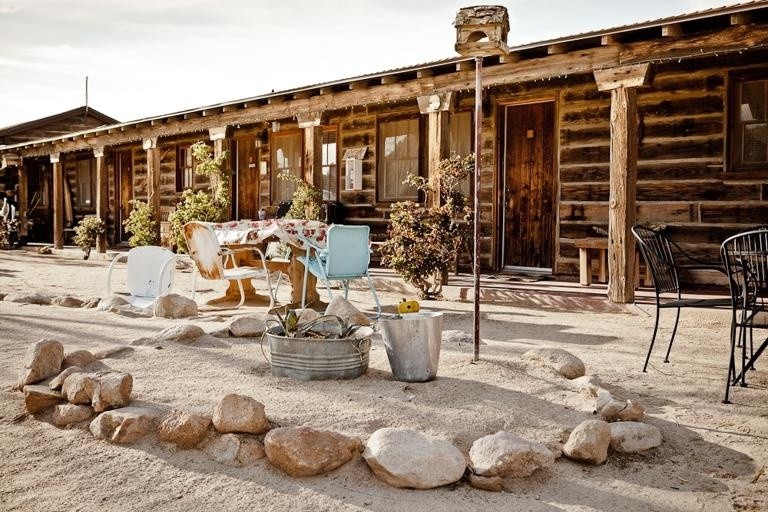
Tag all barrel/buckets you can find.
[258,327,369,380]
[378,311,444,378]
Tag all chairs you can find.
[182,223,277,313]
[716,230,767,402]
[105,244,199,309]
[296,223,381,321]
[627,222,759,382]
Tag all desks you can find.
[197,216,330,317]
[568,232,657,289]
